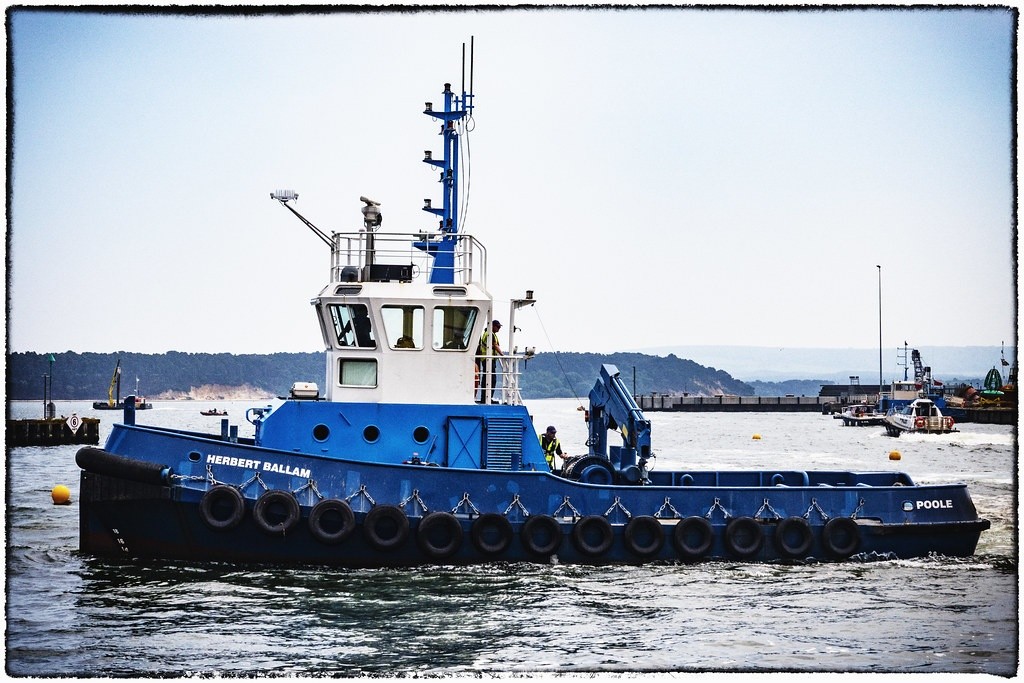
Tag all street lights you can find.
[876,265,883,414]
[41,354,56,420]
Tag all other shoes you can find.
[491,399,500,404]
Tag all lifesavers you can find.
[623,515,665,558]
[198,484,245,532]
[520,514,563,557]
[362,504,409,551]
[470,512,513,556]
[417,512,463,558]
[774,516,817,558]
[945,416,955,428]
[821,516,862,558]
[253,489,300,537]
[915,417,926,428]
[572,514,615,556]
[309,499,356,545]
[723,516,765,559]
[672,515,715,559]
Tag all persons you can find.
[442,333,467,349]
[480,320,504,404]
[338,305,371,347]
[538,426,567,470]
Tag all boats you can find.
[841,404,886,426]
[882,397,959,437]
[71,33,993,563]
[92,358,153,410]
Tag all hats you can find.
[454,333,464,339]
[548,426,557,433]
[493,319,503,327]
